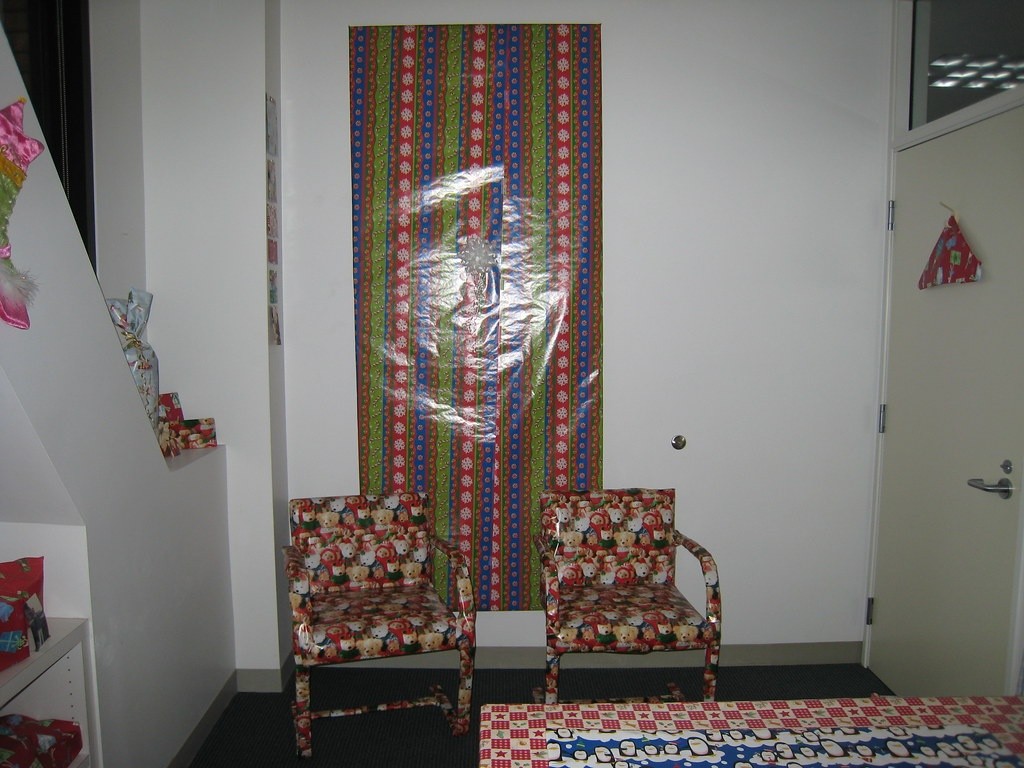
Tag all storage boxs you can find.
[0,596,30,671]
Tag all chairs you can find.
[533,486,722,705]
[280,492,476,761]
